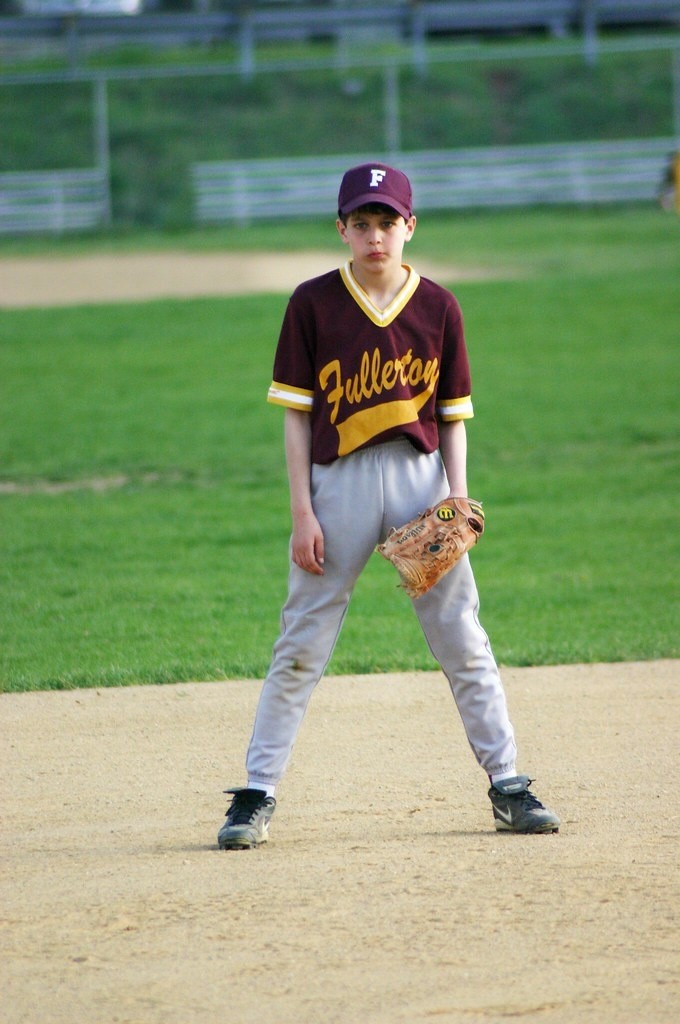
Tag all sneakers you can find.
[488,776,561,834]
[218,787,276,850]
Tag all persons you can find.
[216,165,560,850]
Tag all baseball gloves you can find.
[377,496,486,600]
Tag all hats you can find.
[338,163,412,221]
